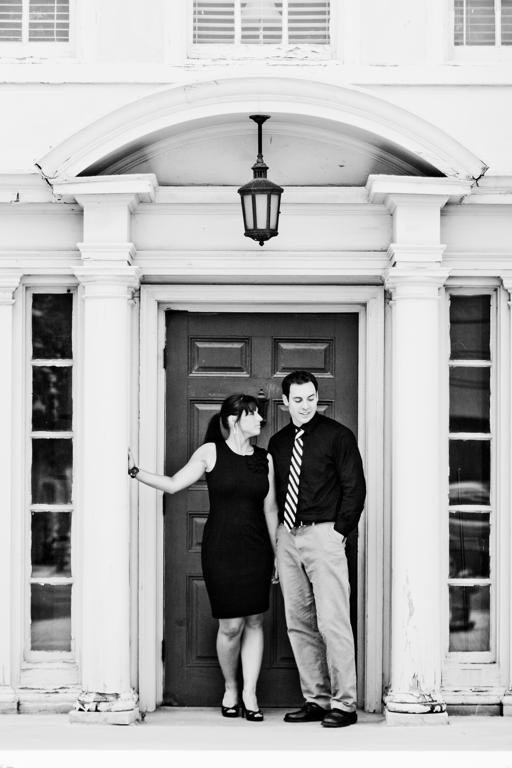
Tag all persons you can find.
[128,394,280,721]
[267,371,366,727]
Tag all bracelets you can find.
[128,467,139,478]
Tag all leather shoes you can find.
[221,702,240,718]
[322,708,360,728]
[284,702,332,723]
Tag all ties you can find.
[282,427,306,534]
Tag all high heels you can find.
[241,694,264,722]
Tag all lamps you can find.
[238,115,284,244]
